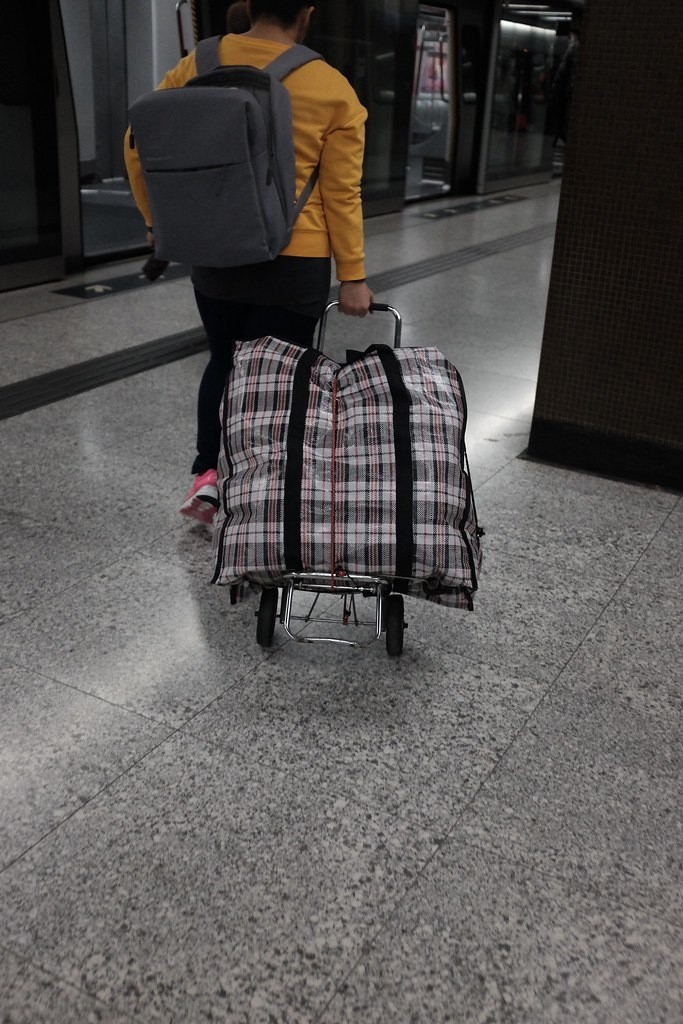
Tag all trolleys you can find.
[252,297,410,659]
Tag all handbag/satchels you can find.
[213,335,485,611]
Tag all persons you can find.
[227,0,252,35]
[123,0,374,523]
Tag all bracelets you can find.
[148,227,152,232]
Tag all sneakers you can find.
[178,468,221,524]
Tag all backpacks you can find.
[126,34,329,267]
